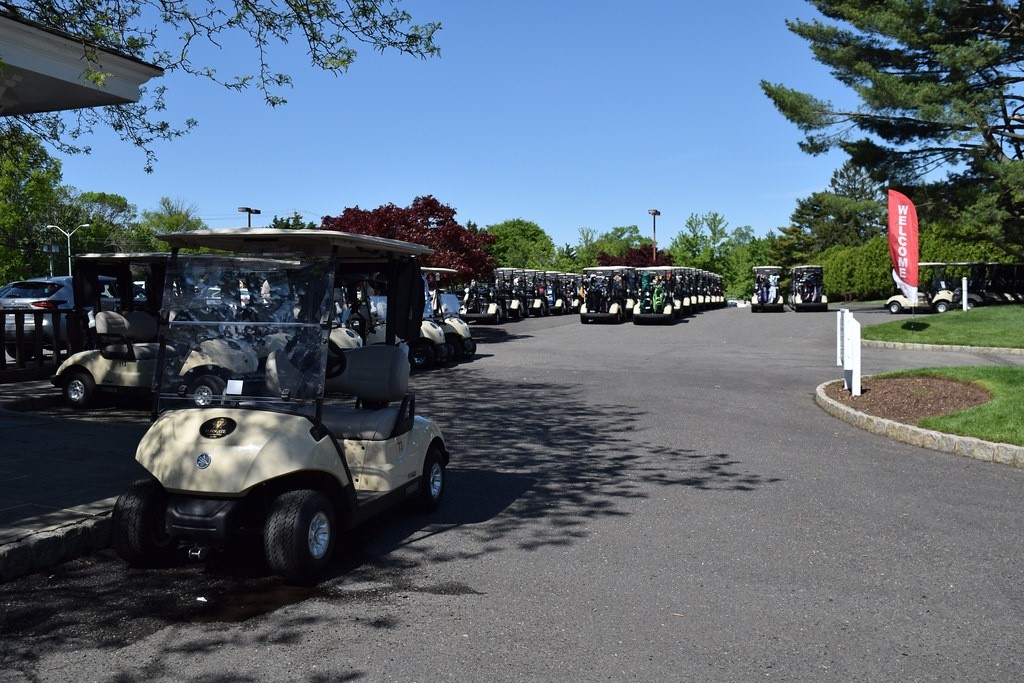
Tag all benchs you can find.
[245,344,410,441]
[96,311,176,359]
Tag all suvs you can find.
[0,275,147,362]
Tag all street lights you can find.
[647,209,661,261]
[46,223,90,276]
[238,207,261,227]
